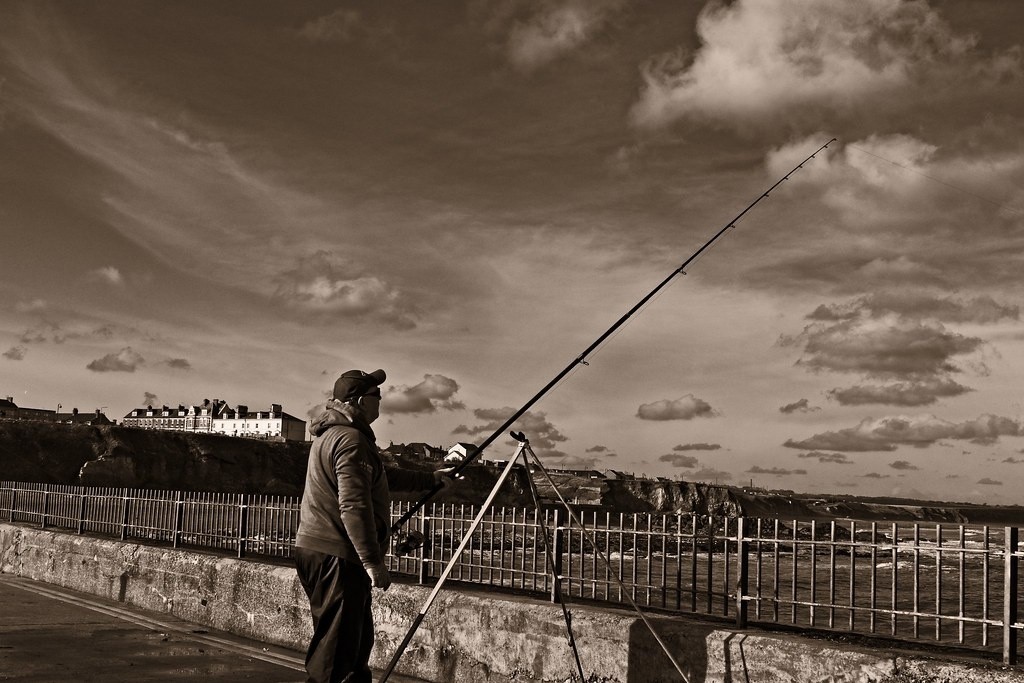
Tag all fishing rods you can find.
[386,137,837,561]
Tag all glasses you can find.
[360,387,382,401]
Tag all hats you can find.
[334,369,386,404]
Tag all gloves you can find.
[420,467,467,494]
[365,564,391,592]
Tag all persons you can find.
[295,370,465,683]
